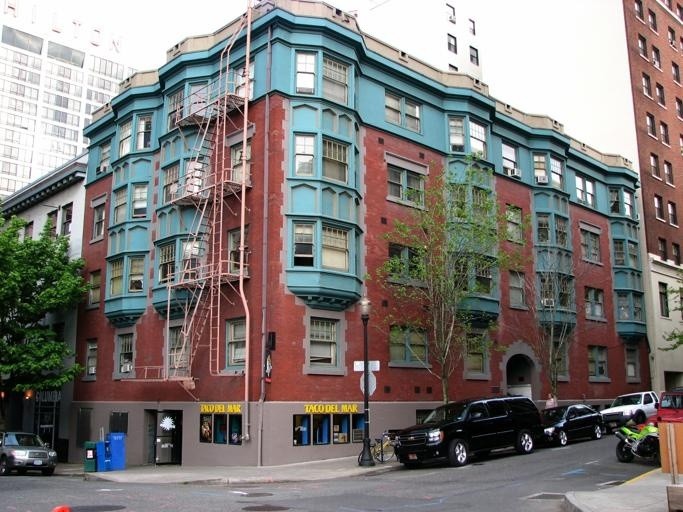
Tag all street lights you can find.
[356,281,375,466]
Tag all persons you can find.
[201,420,211,438]
[545,392,556,409]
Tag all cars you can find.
[1,431,58,476]
[394,391,683,466]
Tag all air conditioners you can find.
[543,298,554,308]
[537,175,548,185]
[510,168,521,179]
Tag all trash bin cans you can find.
[657,391,683,474]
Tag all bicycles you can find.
[358,432,395,465]
